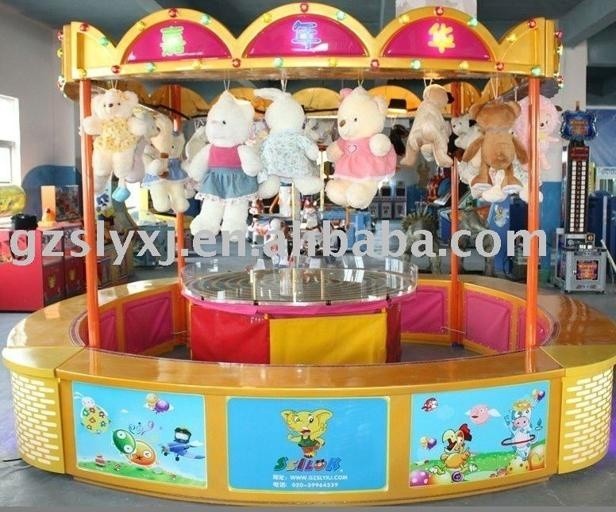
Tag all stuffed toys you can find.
[111,84,560,243]
[111,83,561,244]
[81,87,147,179]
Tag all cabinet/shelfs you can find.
[1,222,84,313]
